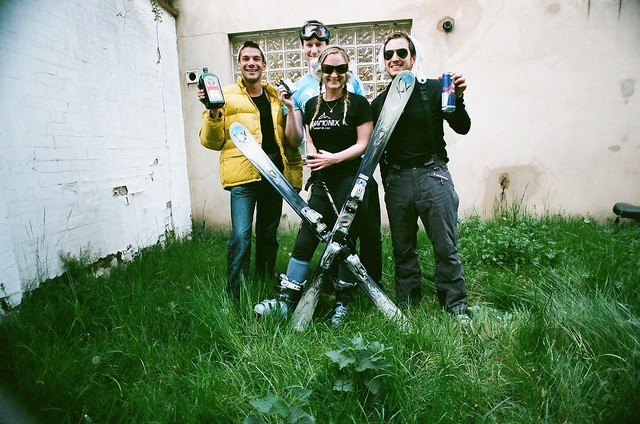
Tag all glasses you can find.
[321,64,348,74]
[299,22,330,41]
[384,49,408,60]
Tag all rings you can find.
[278,93,281,96]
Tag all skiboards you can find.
[229,71,416,335]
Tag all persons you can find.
[371,32,472,326]
[196,39,303,299]
[276,20,383,291]
[253,46,374,324]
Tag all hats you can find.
[237,42,267,64]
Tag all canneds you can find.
[440,71,456,114]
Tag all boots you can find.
[254,272,308,325]
[332,277,358,327]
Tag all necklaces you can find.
[323,93,345,113]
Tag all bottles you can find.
[198,66,225,109]
